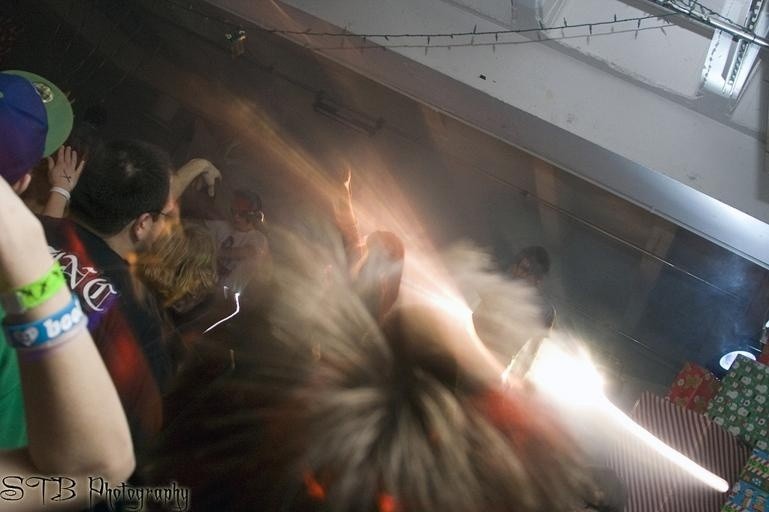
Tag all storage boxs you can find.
[579,355,769,512]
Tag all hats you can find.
[0,70,75,186]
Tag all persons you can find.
[0,68,626,511]
